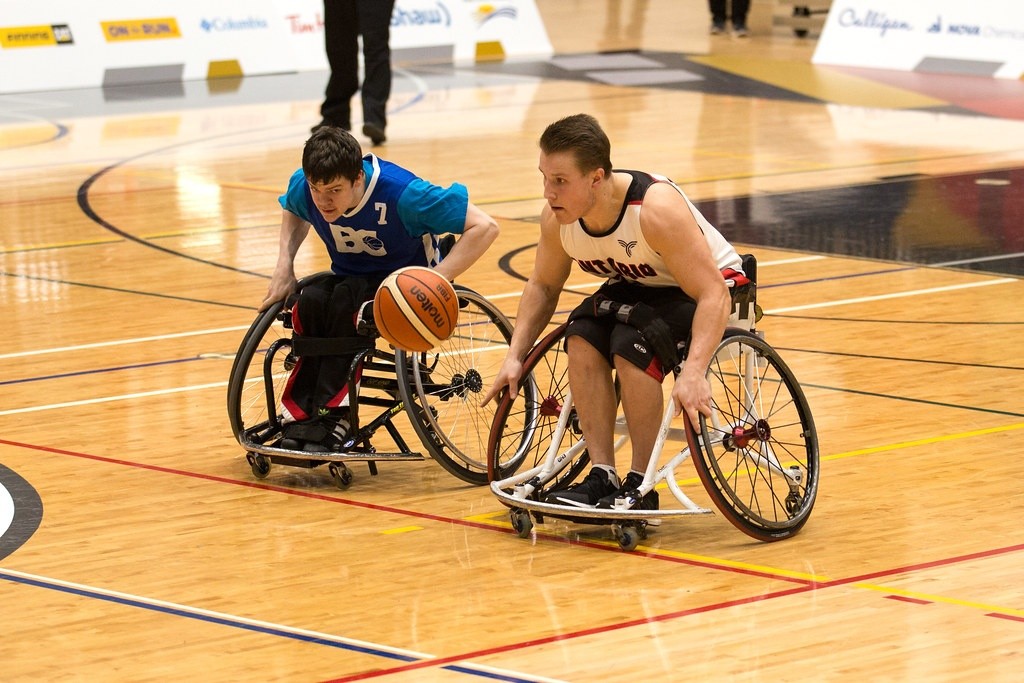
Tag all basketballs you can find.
[373,265,459,352]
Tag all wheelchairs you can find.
[487,254,819,552]
[226,236,539,488]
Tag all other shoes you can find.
[734,24,749,37]
[310,117,351,134]
[361,122,386,144]
[709,22,723,35]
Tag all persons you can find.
[309,0,397,143]
[707,0,752,37]
[256,124,500,457]
[478,112,756,526]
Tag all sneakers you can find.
[596,471,662,525]
[306,408,356,454]
[544,466,622,510]
[280,415,318,452]
